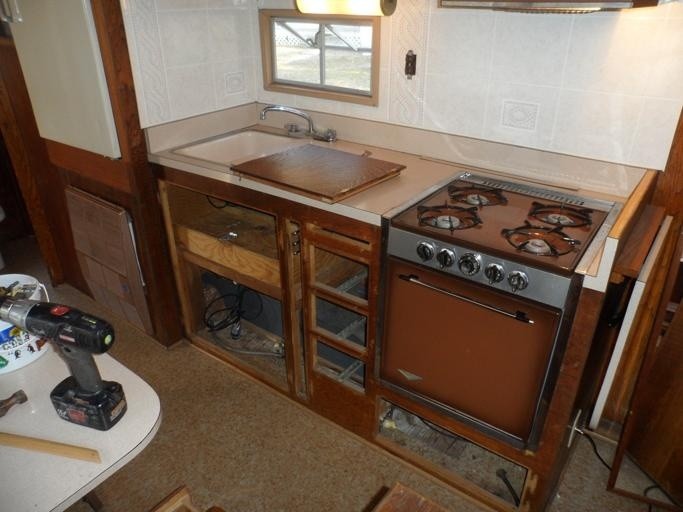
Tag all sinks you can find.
[170,130,307,169]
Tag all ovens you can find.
[376,219,580,449]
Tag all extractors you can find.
[437,1,663,14]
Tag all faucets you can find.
[260,106,314,133]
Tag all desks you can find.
[0,338,163,512]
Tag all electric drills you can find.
[0,295,127,431]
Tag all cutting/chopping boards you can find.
[228,144,406,205]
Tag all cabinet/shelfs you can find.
[149,160,389,443]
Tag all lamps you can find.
[294,0,398,17]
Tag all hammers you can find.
[0,390,101,464]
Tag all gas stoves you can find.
[377,175,612,312]
